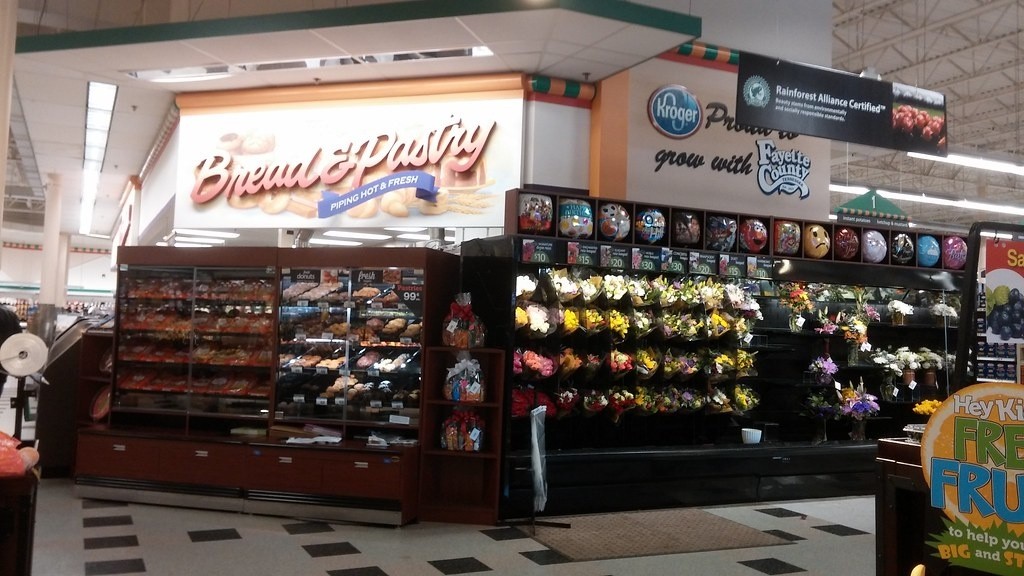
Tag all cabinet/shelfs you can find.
[457,184,974,517]
[416,348,506,523]
[75,245,456,526]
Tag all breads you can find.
[193,130,499,218]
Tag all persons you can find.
[0,302,24,398]
[64,301,111,328]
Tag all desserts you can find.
[280,281,422,399]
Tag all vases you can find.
[891,312,904,325]
[933,316,948,327]
[848,342,862,365]
[814,372,834,385]
[903,369,916,386]
[786,310,804,332]
[924,370,937,387]
[852,419,868,441]
[815,419,828,442]
[822,339,832,362]
[879,370,895,400]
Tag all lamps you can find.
[829,0,1024,241]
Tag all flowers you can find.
[895,345,921,371]
[839,290,881,353]
[512,348,760,381]
[869,346,907,376]
[929,303,958,319]
[798,393,839,421]
[913,399,942,415]
[511,384,762,423]
[775,283,816,315]
[918,347,943,369]
[836,376,881,420]
[887,300,914,316]
[813,309,839,336]
[806,354,838,382]
[516,267,764,346]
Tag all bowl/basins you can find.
[742,428,762,444]
